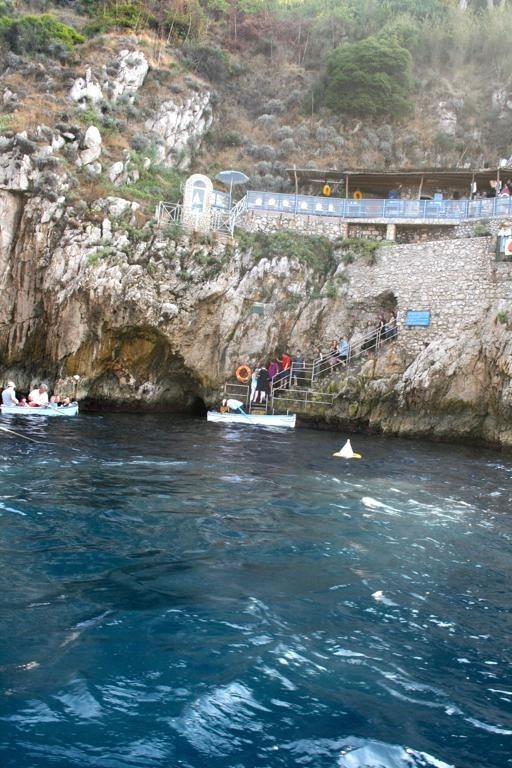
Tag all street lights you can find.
[496,158,508,197]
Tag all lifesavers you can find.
[354,191,362,200]
[323,184,330,197]
[235,365,252,383]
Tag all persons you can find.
[221,398,246,413]
[2,381,71,409]
[458,177,512,200]
[387,187,397,201]
[247,311,397,404]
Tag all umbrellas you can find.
[216,170,249,209]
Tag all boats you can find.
[0,400,82,416]
[204,410,297,429]
